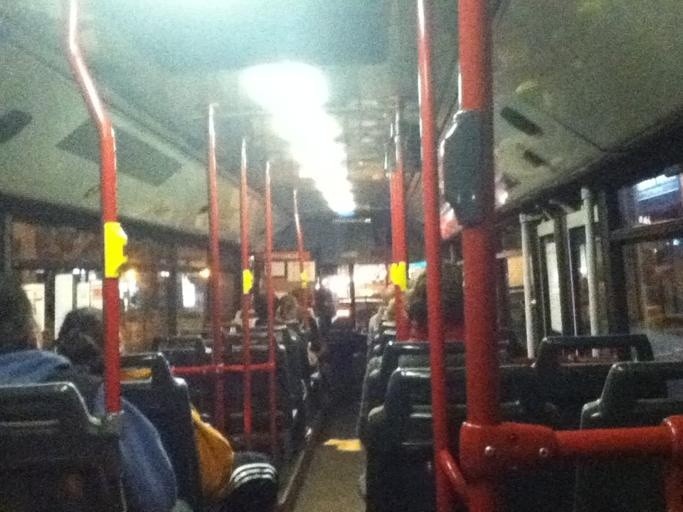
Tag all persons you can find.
[0,268,195,511]
[363,270,405,359]
[496,325,531,359]
[227,275,334,387]
[50,308,280,511]
[357,263,466,499]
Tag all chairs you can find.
[1,353,199,512]
[361,320,465,510]
[499,329,682,511]
[152,324,325,461]
